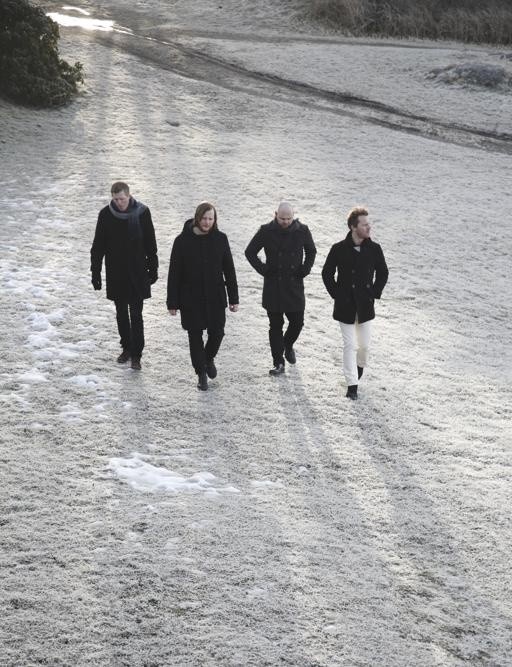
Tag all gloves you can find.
[149,269,159,285]
[91,274,102,290]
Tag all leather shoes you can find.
[269,363,285,374]
[285,350,296,364]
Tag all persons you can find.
[90,181,159,371]
[165,202,240,391]
[243,201,317,375]
[320,206,390,400]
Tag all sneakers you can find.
[118,351,131,363]
[197,374,208,390]
[357,367,364,379]
[132,359,142,370]
[205,363,217,379]
[346,385,358,400]
